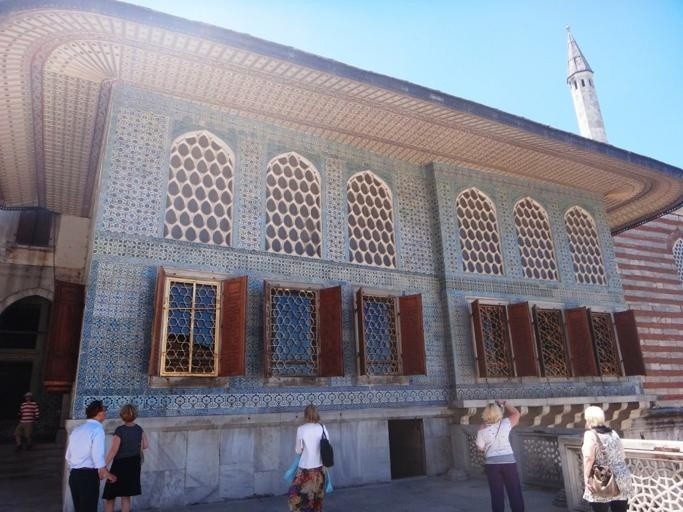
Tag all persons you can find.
[65,400,118,512]
[581,406,632,512]
[476,398,525,512]
[288,405,330,512]
[14,392,40,450]
[99,405,149,512]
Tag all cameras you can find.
[495,404,504,409]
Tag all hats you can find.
[24,392,32,396]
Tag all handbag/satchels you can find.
[582,429,620,498]
[318,422,334,468]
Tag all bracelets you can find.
[503,400,507,405]
